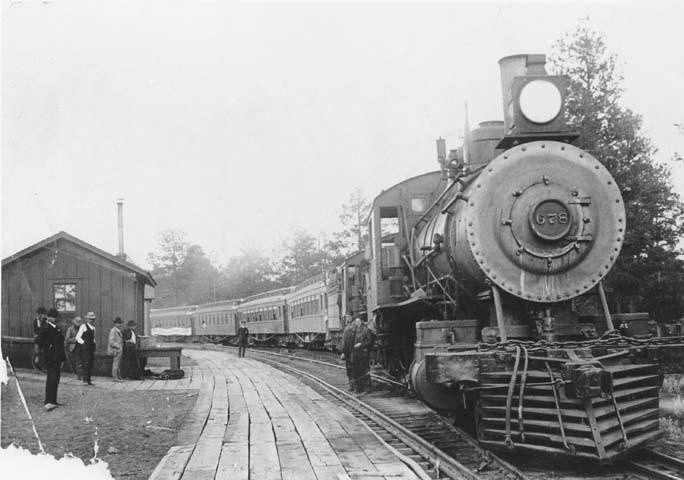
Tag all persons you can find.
[63,316,82,380]
[37,308,65,409]
[122,319,144,380]
[106,317,126,382]
[74,312,97,386]
[32,307,47,364]
[340,311,357,392]
[235,321,249,358]
[352,317,373,392]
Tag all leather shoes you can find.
[45,403,57,410]
[77,377,147,385]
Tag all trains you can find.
[148,52,684,471]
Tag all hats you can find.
[37,307,137,327]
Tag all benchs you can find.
[137,345,184,378]
